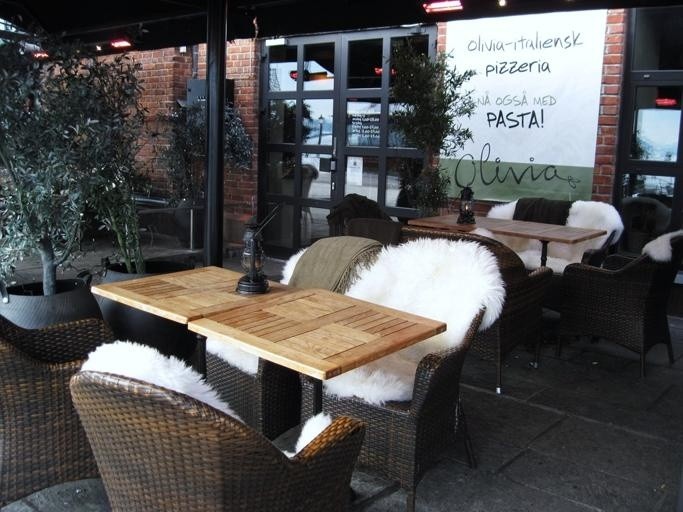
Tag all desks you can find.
[188,285,447,512]
[92,266,298,325]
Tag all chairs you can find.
[1,316,114,512]
[327,238,509,512]
[270,164,318,223]
[280,236,380,300]
[68,340,363,512]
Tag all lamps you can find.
[238,201,283,293]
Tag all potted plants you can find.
[0,38,101,332]
[70,42,199,362]
[149,101,255,250]
